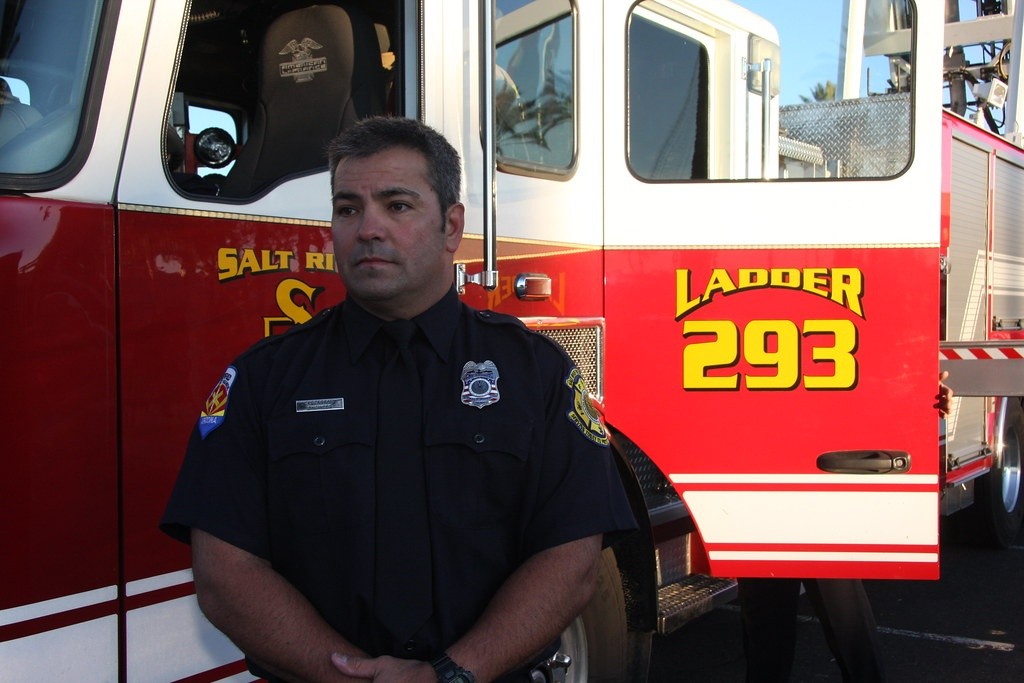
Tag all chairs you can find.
[218,0,400,200]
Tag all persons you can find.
[154,113,641,682]
[737,578,889,683]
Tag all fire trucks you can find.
[1,0,1023,681]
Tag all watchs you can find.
[429,652,476,683]
